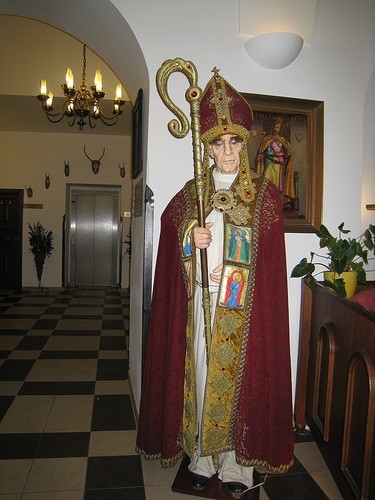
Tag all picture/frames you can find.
[239,92,324,233]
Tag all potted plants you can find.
[290,221,375,300]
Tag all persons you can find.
[134,68,296,494]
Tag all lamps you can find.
[242,32,305,71]
[35,43,125,130]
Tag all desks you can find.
[294,276,375,500]
